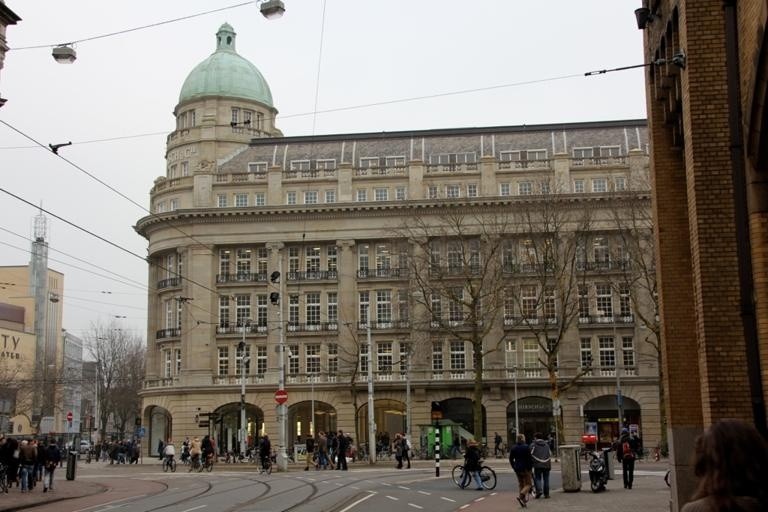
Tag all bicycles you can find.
[162,453,214,472]
[452,453,496,490]
[665,470,671,487]
[525,471,536,502]
[254,447,272,475]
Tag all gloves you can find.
[616,456,622,463]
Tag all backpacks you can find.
[622,439,635,459]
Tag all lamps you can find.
[52,42,77,65]
[259,0,285,20]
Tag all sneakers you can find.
[535,491,552,499]
[304,464,349,472]
[475,485,484,490]
[457,483,465,490]
[623,480,633,489]
[396,460,411,470]
[516,492,529,509]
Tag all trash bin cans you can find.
[601,448,615,479]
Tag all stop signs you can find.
[274,391,288,404]
[66,412,73,421]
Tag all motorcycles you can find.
[582,450,608,492]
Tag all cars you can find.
[81,441,95,454]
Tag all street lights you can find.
[307,372,315,438]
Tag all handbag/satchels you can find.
[407,439,412,449]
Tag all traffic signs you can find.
[199,413,222,427]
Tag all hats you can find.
[620,427,630,435]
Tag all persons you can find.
[158,435,216,472]
[456,437,483,490]
[286,430,355,471]
[616,427,636,490]
[224,434,277,472]
[366,431,389,461]
[528,432,554,499]
[493,431,505,455]
[1,436,68,496]
[680,417,768,512]
[86,439,140,464]
[393,433,411,469]
[508,434,533,506]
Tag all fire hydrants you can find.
[655,448,660,460]
